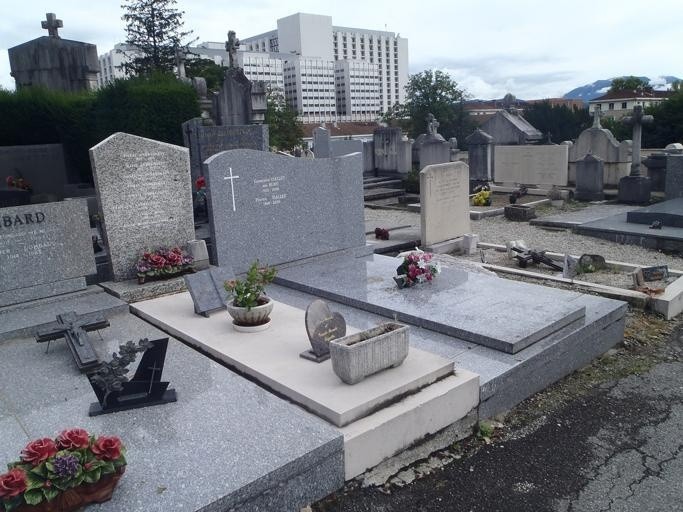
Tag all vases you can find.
[223,294,275,331]
[7,461,128,511]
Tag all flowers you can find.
[221,256,282,312]
[0,428,128,511]
[131,242,199,283]
[392,246,441,288]
[193,176,207,201]
[470,187,491,208]
[4,175,35,196]
